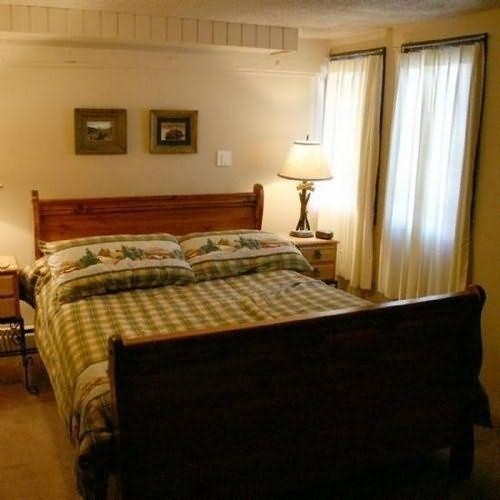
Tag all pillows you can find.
[176,225,315,284]
[35,231,198,300]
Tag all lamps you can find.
[277,132,336,239]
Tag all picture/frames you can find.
[73,106,129,156]
[148,108,199,154]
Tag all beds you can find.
[22,181,487,497]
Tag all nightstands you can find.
[2,254,23,316]
[289,236,340,288]
